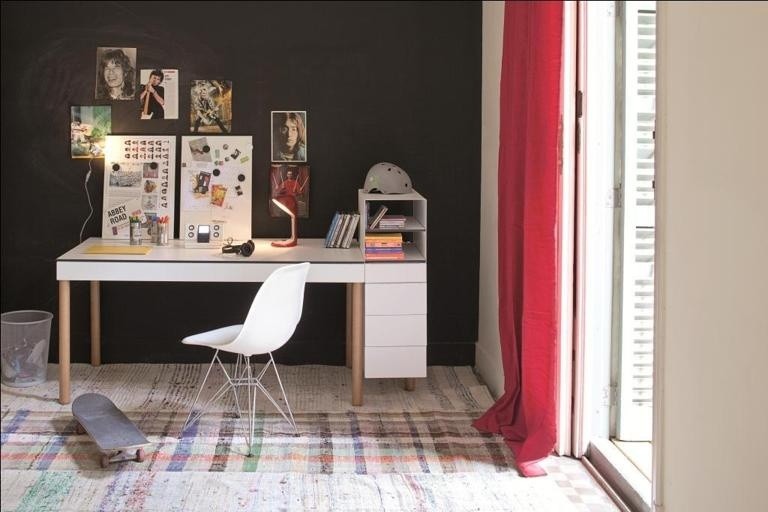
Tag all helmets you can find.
[364,162,412,194]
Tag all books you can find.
[81,245,154,254]
[366,205,407,260]
[323,209,361,248]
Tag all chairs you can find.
[177,262,313,456]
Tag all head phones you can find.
[222,240,254,256]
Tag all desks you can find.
[55,238,365,406]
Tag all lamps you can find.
[272,195,298,247]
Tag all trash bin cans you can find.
[0,310,53,388]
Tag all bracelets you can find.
[152,90,156,95]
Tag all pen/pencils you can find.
[129,215,169,223]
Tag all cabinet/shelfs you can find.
[358,189,428,391]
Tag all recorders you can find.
[184,223,224,249]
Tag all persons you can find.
[71,117,89,145]
[97,49,135,101]
[273,170,304,213]
[139,70,165,118]
[199,176,209,186]
[193,84,227,134]
